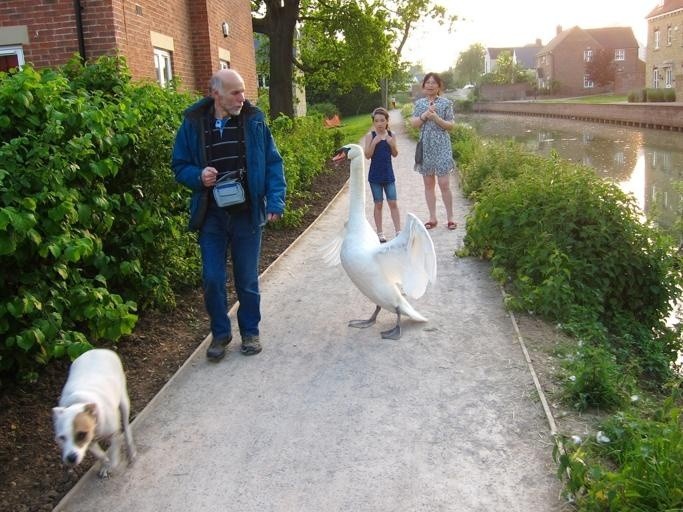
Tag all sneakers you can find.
[206,334,232,361]
[240,335,262,355]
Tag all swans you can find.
[317,143,437,341]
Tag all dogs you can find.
[51,348,138,481]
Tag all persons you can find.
[392,96,395,108]
[173,66,289,361]
[415,73,459,230]
[364,108,401,245]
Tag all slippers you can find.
[448,221,456,229]
[425,221,438,229]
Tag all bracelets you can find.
[419,112,426,123]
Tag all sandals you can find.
[377,233,386,242]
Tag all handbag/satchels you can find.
[210,177,246,208]
[415,142,423,163]
